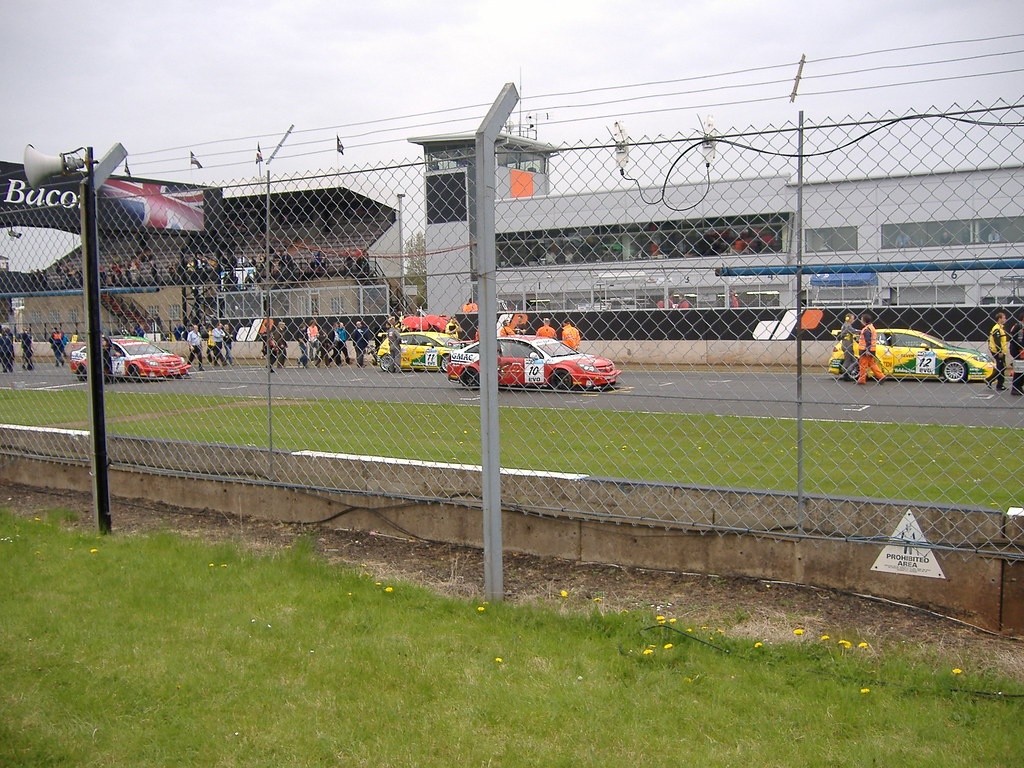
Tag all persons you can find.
[840,313,887,386]
[261,318,288,373]
[48,326,69,367]
[110,344,122,357]
[20,327,35,371]
[115,323,145,338]
[717,292,738,307]
[295,297,581,373]
[0,326,15,372]
[101,331,117,385]
[187,324,206,371]
[55,247,369,289]
[172,318,236,367]
[983,313,1024,396]
[656,289,688,308]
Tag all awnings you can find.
[809,272,878,287]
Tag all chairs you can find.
[42,231,385,291]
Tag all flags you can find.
[125,164,132,177]
[192,152,203,169]
[256,145,262,164]
[337,138,345,156]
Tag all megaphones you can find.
[24,144,83,190]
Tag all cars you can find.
[446,335,622,394]
[827,329,996,384]
[376,331,468,373]
[69,335,191,383]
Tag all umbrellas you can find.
[426,315,448,330]
[436,314,451,320]
[402,316,430,330]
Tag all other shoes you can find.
[199,367,205,371]
[1010,390,1024,395]
[55,362,60,366]
[214,364,220,366]
[844,377,854,382]
[266,365,275,373]
[62,360,64,366]
[222,363,230,366]
[983,378,991,389]
[877,376,888,386]
[1,369,9,373]
[855,381,866,385]
[996,386,1009,391]
